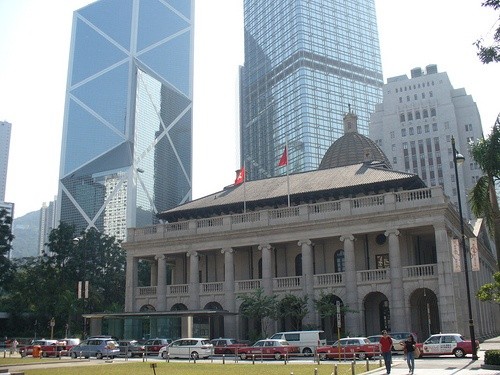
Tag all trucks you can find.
[270,330,327,357]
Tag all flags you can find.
[235,166,244,185]
[279,146,287,166]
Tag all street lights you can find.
[450,134,479,361]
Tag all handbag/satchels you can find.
[413,347,421,358]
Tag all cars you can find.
[19,335,121,360]
[387,332,420,351]
[314,337,383,361]
[237,339,301,361]
[366,335,383,344]
[413,333,481,359]
[142,337,172,355]
[210,337,247,354]
[158,337,215,360]
[117,340,149,358]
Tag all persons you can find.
[405,334,417,375]
[379,330,393,374]
[13,339,19,354]
